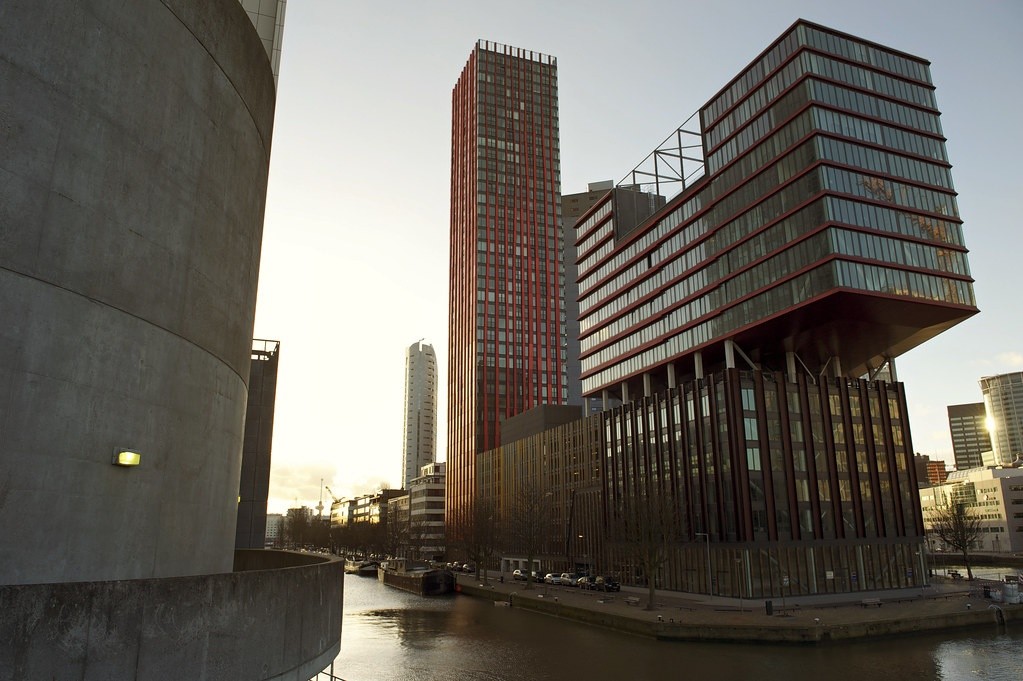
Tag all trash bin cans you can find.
[765,601,772,615]
[984,586,990,598]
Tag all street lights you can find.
[579,535,587,590]
[695,532,712,598]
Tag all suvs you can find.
[595,575,620,592]
[513,568,528,581]
[560,572,579,587]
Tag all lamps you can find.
[111,446,142,467]
[238,495,241,503]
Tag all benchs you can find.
[564,589,575,593]
[598,594,618,602]
[580,591,595,596]
[1005,575,1019,584]
[656,603,664,607]
[892,597,918,604]
[625,597,642,606]
[947,569,965,579]
[679,605,697,612]
[859,597,884,609]
[713,608,753,614]
[455,570,560,592]
[773,607,802,614]
[814,604,843,610]
[928,595,953,601]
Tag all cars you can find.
[305,544,475,574]
[543,572,561,585]
[578,576,596,591]
[531,570,546,583]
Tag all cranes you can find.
[325,485,345,504]
